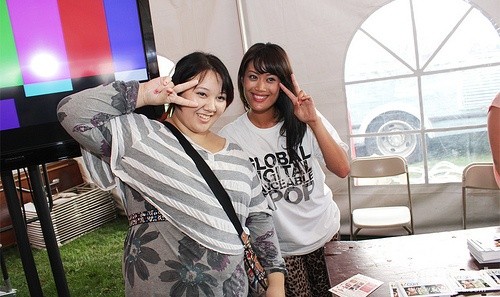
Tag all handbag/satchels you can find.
[243,246,268,296]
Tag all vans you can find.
[345,55,500,167]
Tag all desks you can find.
[324,226,500,297]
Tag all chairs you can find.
[348,155,414,241]
[461,162,500,229]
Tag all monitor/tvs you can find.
[0,0,167,173]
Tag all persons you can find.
[486,91,500,190]
[216,42,351,297]
[57,52,289,297]
